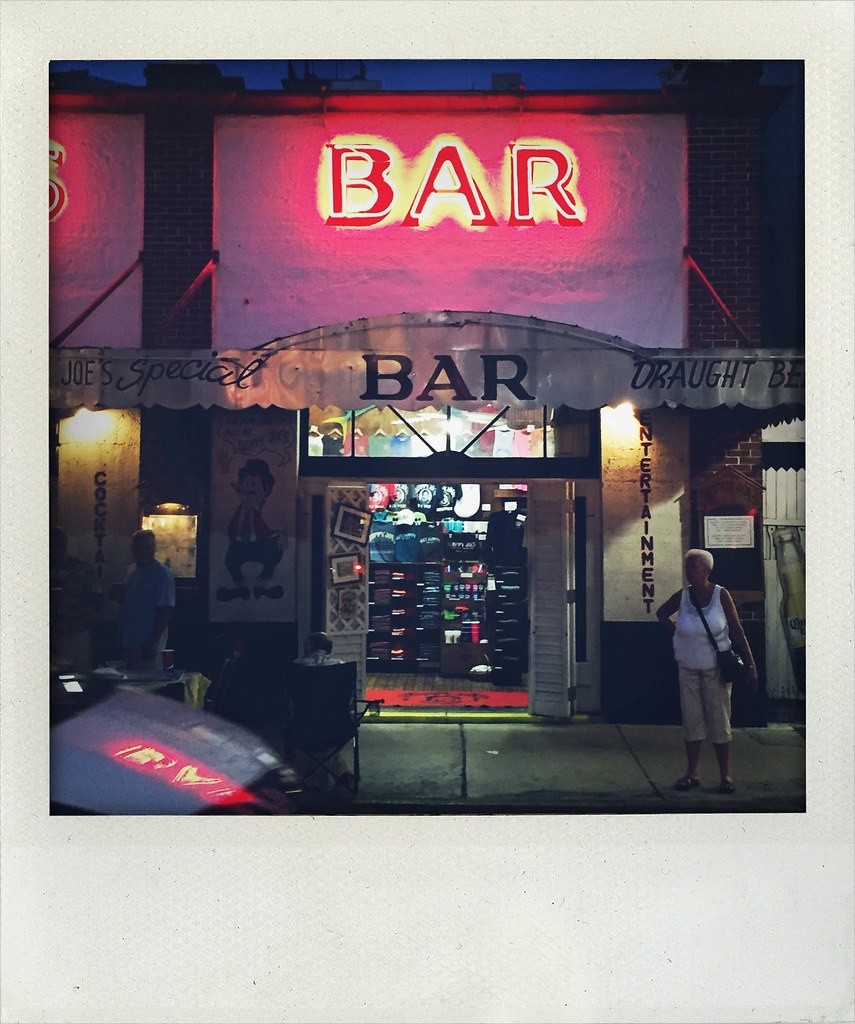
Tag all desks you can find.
[112,666,211,710]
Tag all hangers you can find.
[308,424,552,437]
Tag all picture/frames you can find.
[333,504,372,546]
[329,552,363,586]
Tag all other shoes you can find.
[718,777,735,794]
[675,775,700,791]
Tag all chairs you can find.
[284,661,384,798]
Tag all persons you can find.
[51,526,103,668]
[50,627,112,725]
[114,530,176,674]
[656,548,758,795]
[288,632,354,794]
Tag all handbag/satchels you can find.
[717,645,749,683]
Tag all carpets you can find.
[365,687,529,706]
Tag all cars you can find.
[50,654,307,815]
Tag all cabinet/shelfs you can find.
[488,566,524,685]
[370,562,440,674]
[441,559,487,678]
